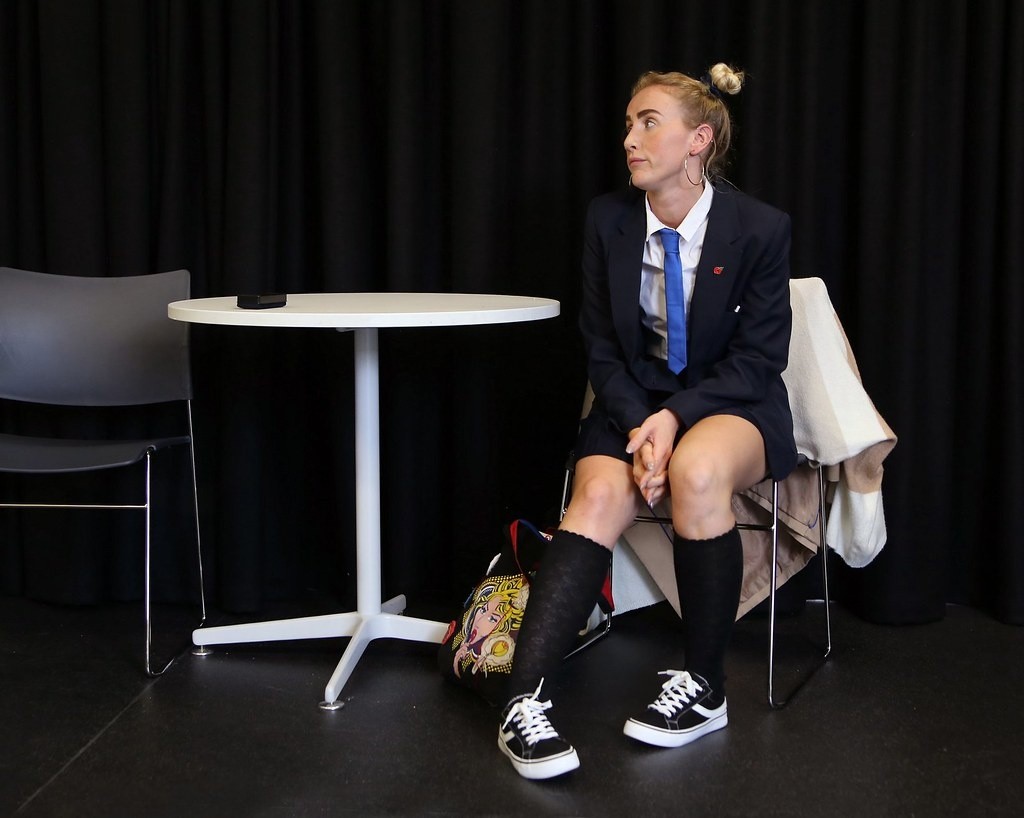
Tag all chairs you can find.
[0,267,209,678]
[559,277,899,713]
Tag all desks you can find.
[166,294,561,717]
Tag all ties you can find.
[660,230,686,375]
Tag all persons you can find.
[499,64,797,781]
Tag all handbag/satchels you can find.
[441,519,615,694]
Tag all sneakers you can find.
[498,706,580,780]
[624,671,727,746]
